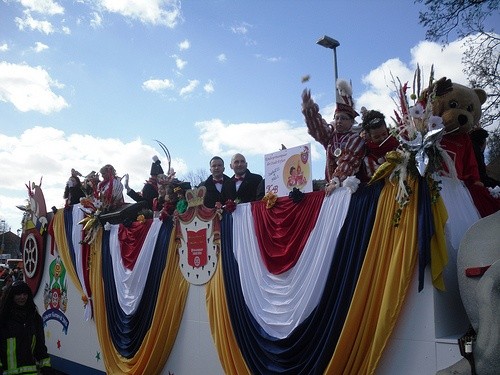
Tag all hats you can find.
[157,171,176,185]
[101,164,114,174]
[167,178,180,184]
[150,155,164,176]
[334,102,360,119]
[173,182,191,192]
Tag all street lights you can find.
[315,34,341,105]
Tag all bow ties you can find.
[212,180,223,185]
[233,177,244,182]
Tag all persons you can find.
[197,157,232,207]
[362,110,401,181]
[125,160,191,210]
[63,176,85,205]
[0,265,26,301]
[300,87,365,193]
[83,176,95,199]
[0,280,53,375]
[221,153,263,206]
[98,165,123,210]
[472,127,500,188]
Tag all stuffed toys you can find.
[423,76,488,187]
